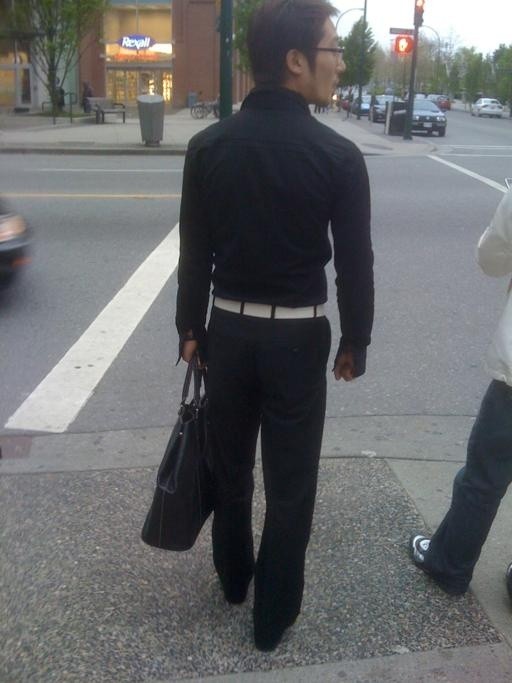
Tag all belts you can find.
[214,296,327,319]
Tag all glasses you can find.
[309,46,345,64]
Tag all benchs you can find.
[84,95,125,122]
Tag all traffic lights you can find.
[396,36,412,56]
[413,0,424,27]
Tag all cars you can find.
[334,91,450,138]
[472,98,505,118]
[1,204,32,285]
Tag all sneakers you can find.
[410,533,431,570]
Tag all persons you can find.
[56,77,64,112]
[314,105,329,113]
[174,0,377,651]
[411,173,512,607]
[83,80,93,113]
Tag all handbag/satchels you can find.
[141,354,215,551]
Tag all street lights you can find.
[336,7,365,30]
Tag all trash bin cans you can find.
[137,95,164,146]
[384,101,408,136]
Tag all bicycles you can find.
[190,97,220,119]
